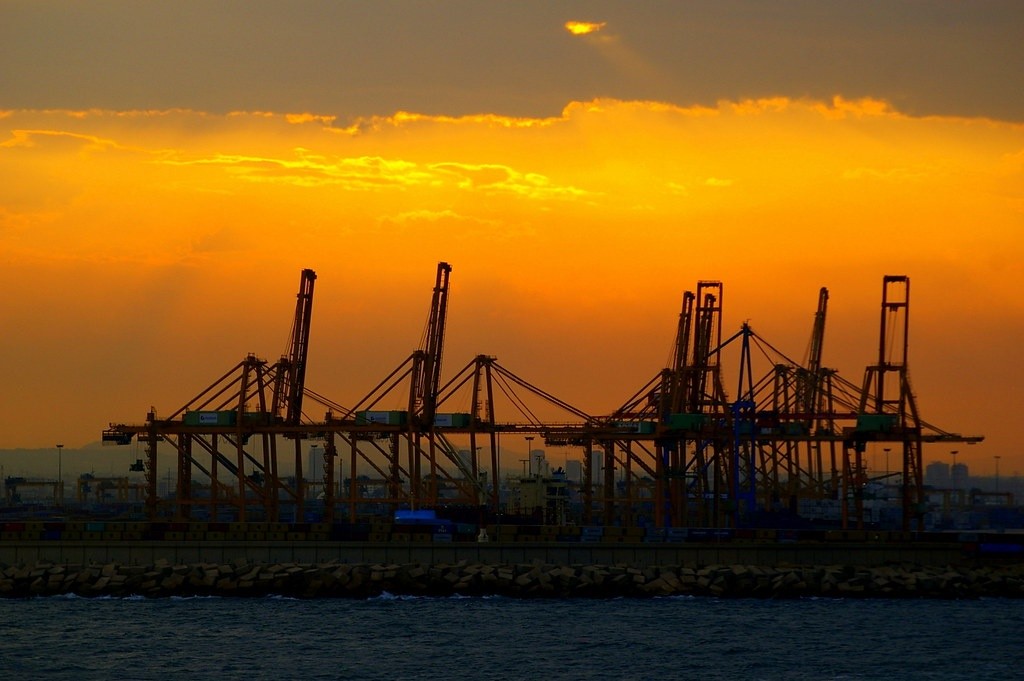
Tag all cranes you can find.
[95,260,988,533]
